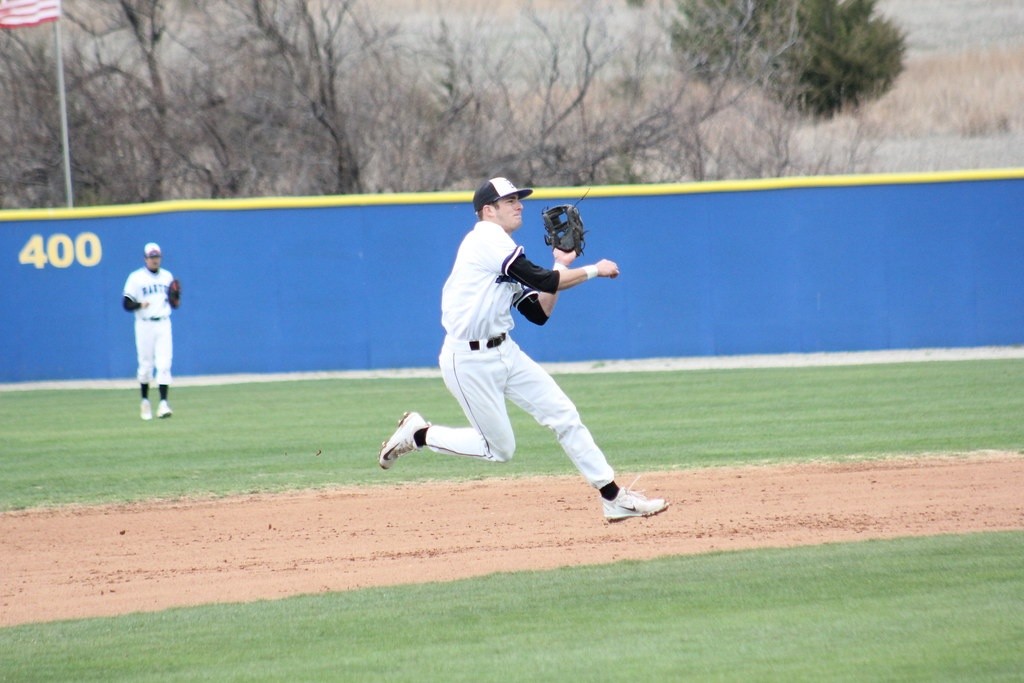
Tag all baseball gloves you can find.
[543,204,584,257]
[168,280,180,308]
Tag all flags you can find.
[0,0,59,28]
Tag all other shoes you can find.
[156,400,172,418]
[140,398,152,420]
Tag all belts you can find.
[151,317,160,320]
[469,333,506,351]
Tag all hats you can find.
[143,243,161,258]
[473,177,533,212]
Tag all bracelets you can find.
[581,265,598,280]
[553,263,567,272]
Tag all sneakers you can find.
[378,412,431,470]
[601,475,668,522]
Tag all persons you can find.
[379,178,668,523]
[123,243,180,420]
